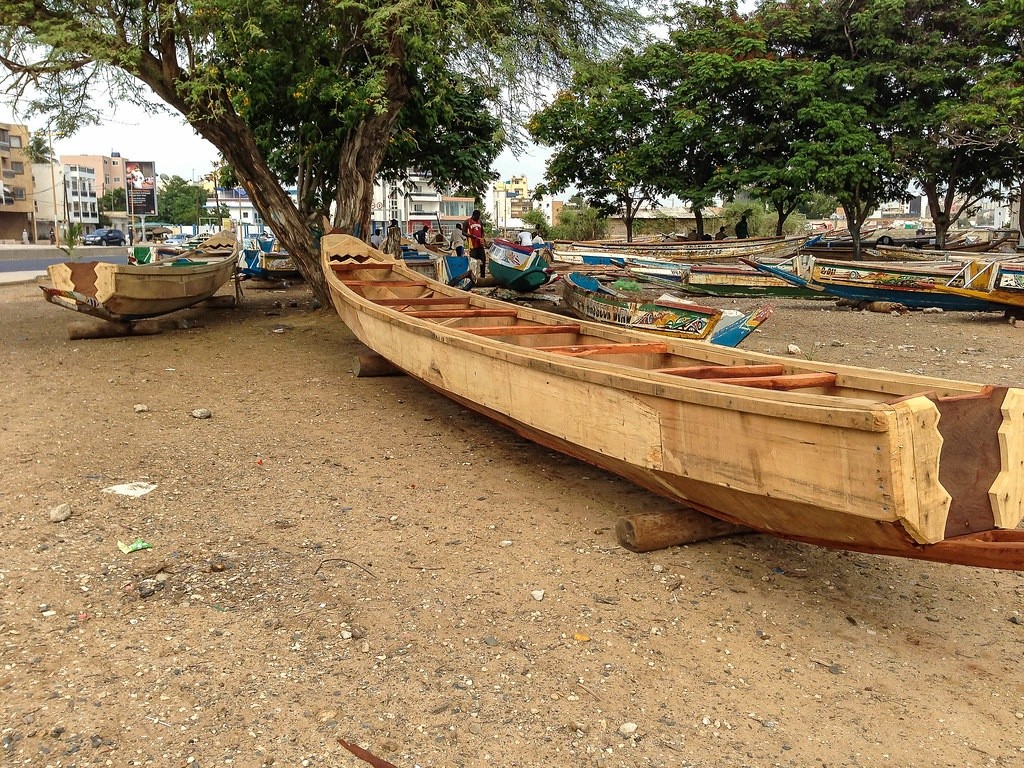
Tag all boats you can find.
[34,229,239,323]
[237,230,301,279]
[549,219,1023,321]
[319,233,1024,574]
[487,236,561,293]
[561,272,778,348]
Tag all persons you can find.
[715,226,728,240]
[128,227,133,246]
[448,223,466,257]
[735,215,749,239]
[430,233,449,243]
[371,229,383,247]
[413,225,429,246]
[49,228,56,244]
[662,229,711,242]
[22,229,28,245]
[515,230,544,246]
[462,210,486,278]
[383,219,402,259]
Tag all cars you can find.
[82,228,127,247]
[163,232,194,244]
